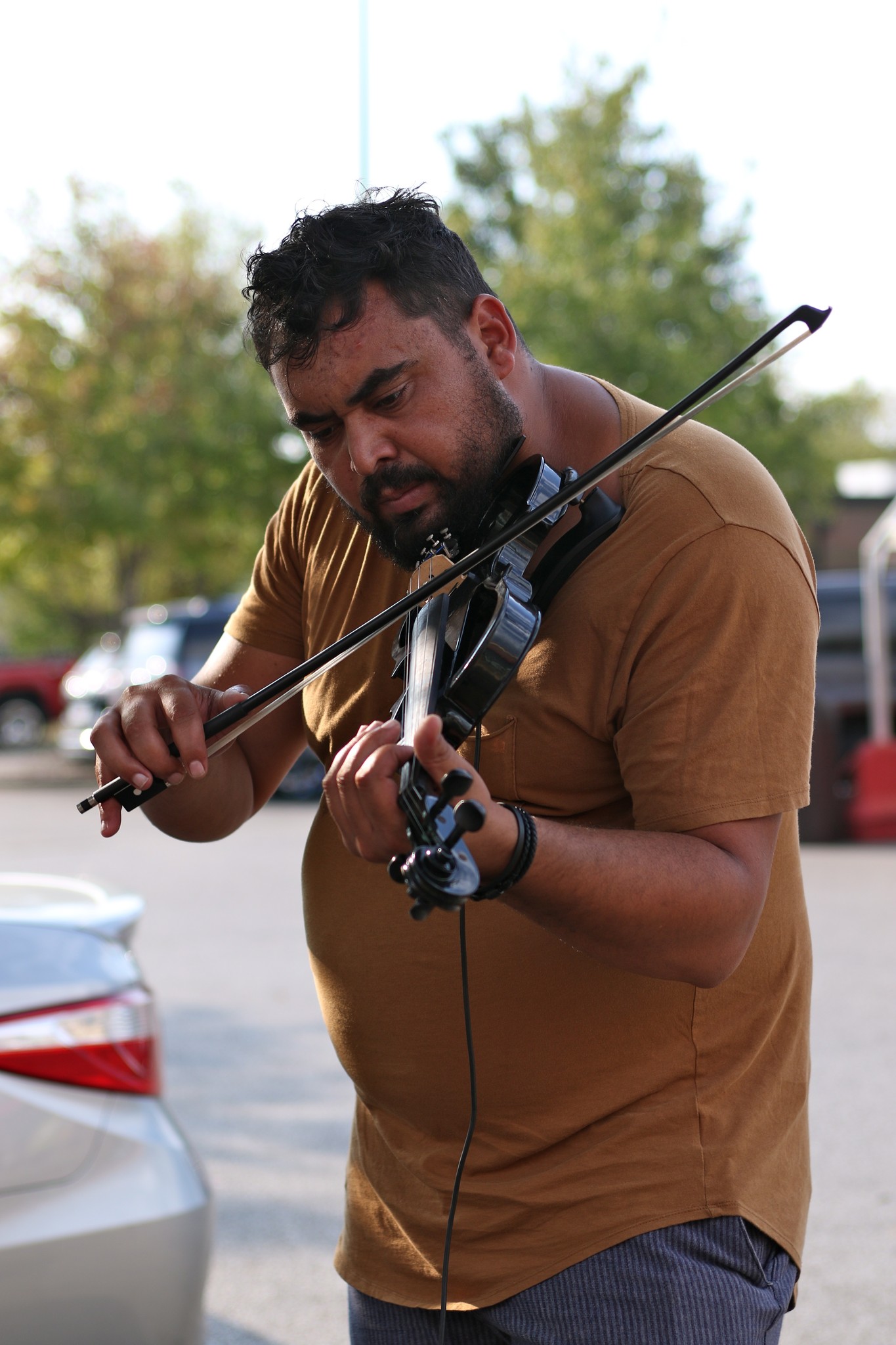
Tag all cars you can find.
[0,603,324,808]
[0,873,210,1344]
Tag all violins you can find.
[389,452,580,923]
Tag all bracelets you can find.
[470,802,538,901]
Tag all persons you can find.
[91,181,822,1345]
